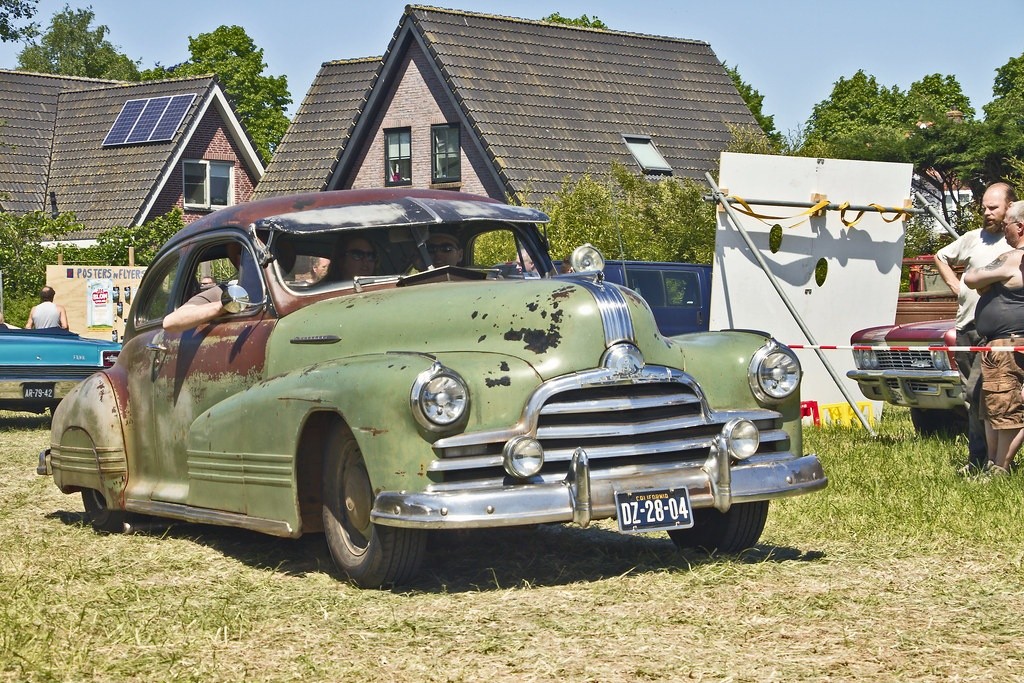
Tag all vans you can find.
[487,259,714,337]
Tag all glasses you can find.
[1002,222,1019,229]
[426,242,460,253]
[344,249,380,262]
[201,283,212,285]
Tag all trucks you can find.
[894,256,965,326]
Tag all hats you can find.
[428,224,463,248]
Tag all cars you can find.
[35,182,829,594]
[1,322,122,413]
[847,317,963,438]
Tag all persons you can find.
[933,183,1024,483]
[515,249,537,274]
[415,225,464,272]
[162,235,297,332]
[304,257,331,284]
[336,234,377,279]
[561,255,573,273]
[25,286,68,330]
[201,277,216,285]
[0,313,8,329]
